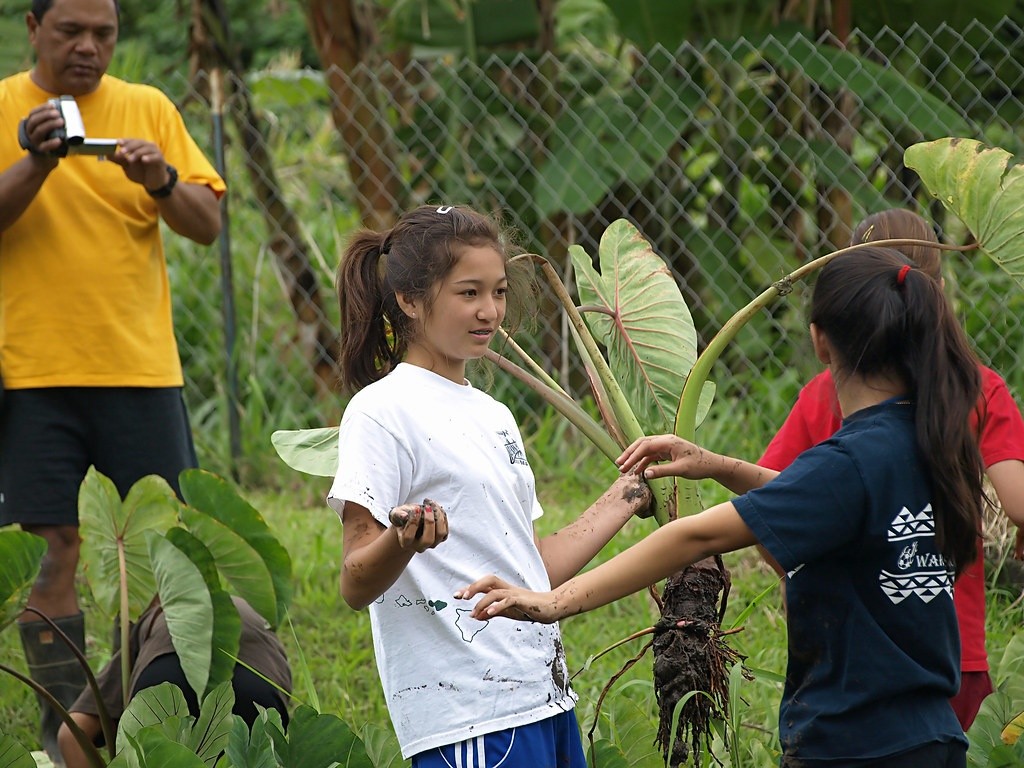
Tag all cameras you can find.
[46,95,118,157]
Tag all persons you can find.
[325,203,1024,768]
[0,0,295,768]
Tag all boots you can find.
[18,610,91,767]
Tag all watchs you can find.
[145,164,179,199]
